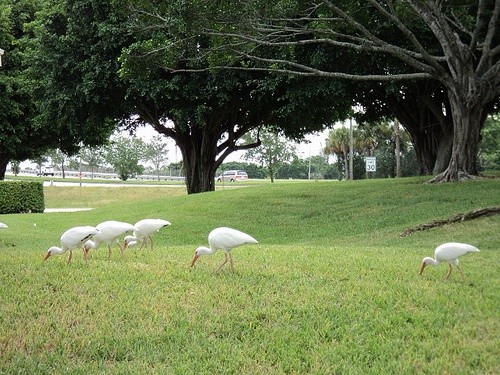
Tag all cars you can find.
[217,170,248,182]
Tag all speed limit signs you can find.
[366,157,376,171]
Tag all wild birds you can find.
[44,225,102,264]
[419,242,480,281]
[121,219,172,252]
[82,220,139,259]
[190,227,259,274]
[127,235,147,248]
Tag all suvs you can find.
[37,166,54,177]
